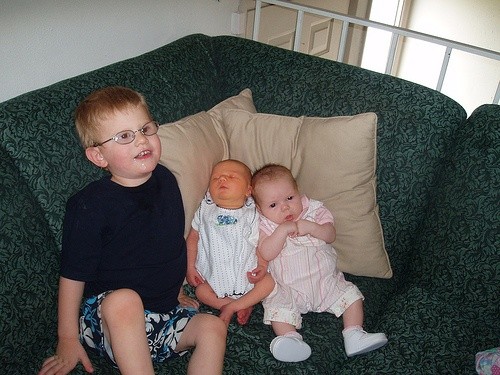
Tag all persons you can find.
[37,85,228,375]
[184,157,274,326]
[249,163,388,362]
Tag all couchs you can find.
[0,33,500,375]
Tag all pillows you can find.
[223,108,392,280]
[156,88,258,241]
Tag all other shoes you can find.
[270,332,311,362]
[342,326,388,357]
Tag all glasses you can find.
[100,120,160,145]
